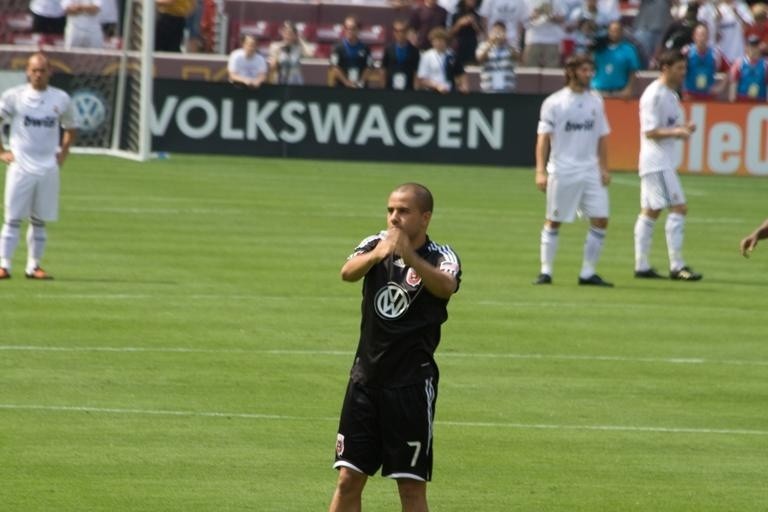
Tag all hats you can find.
[749,35,761,44]
[280,21,299,39]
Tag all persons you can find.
[228,34,267,85]
[381,16,420,91]
[563,0,610,47]
[416,28,473,93]
[729,36,768,101]
[329,17,374,89]
[0,54,80,280]
[635,51,703,283]
[576,21,598,59]
[268,18,301,84]
[59,0,108,48]
[744,3,768,48]
[475,21,520,92]
[591,23,639,97]
[414,0,447,32]
[741,219,768,259]
[679,25,731,99]
[330,182,463,512]
[648,2,705,77]
[446,1,488,65]
[29,0,66,33]
[713,0,754,61]
[531,53,613,287]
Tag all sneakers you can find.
[578,274,614,287]
[634,269,665,279]
[0,269,10,279]
[25,267,50,279]
[532,274,551,285]
[670,268,702,280]
